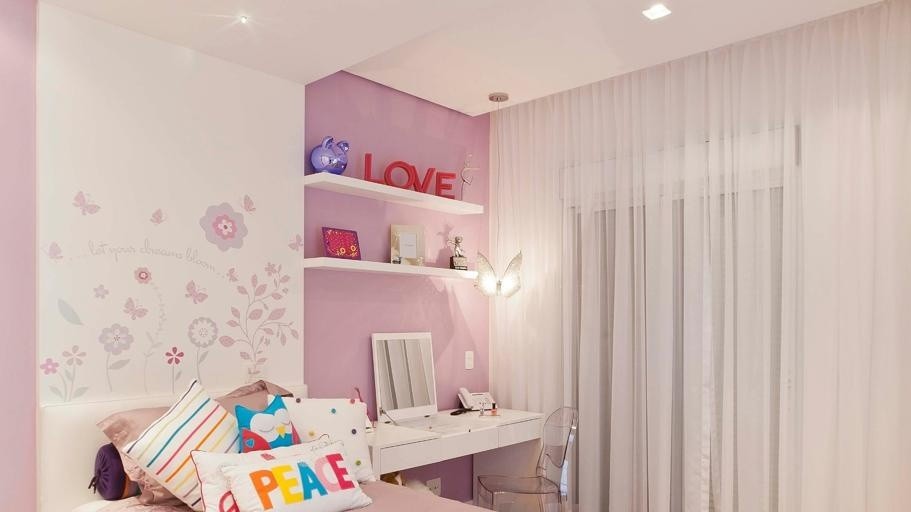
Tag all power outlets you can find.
[426,478,441,496]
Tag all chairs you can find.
[477,406,579,512]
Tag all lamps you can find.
[473,92,522,299]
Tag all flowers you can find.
[200,202,247,251]
[43,262,300,402]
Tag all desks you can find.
[368,408,545,512]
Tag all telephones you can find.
[457,387,498,410]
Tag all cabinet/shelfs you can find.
[304,172,483,279]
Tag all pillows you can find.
[87,379,373,511]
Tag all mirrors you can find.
[372,332,437,423]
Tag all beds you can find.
[41,391,502,512]
